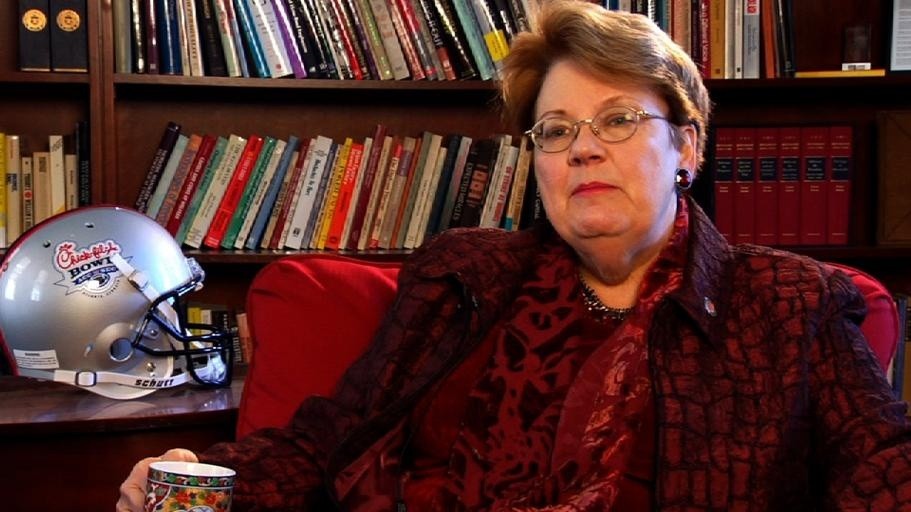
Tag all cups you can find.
[142,460,237,512]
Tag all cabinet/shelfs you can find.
[104,2,911,378]
[0,0,104,261]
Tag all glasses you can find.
[522,104,669,155]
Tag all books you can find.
[712,125,853,245]
[0,134,91,248]
[132,121,546,253]
[112,0,532,82]
[187,307,254,364]
[601,1,795,79]
[15,0,88,73]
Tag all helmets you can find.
[0,202,237,402]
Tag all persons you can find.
[110,2,910,512]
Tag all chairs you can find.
[226,252,907,442]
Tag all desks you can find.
[2,379,242,512]
